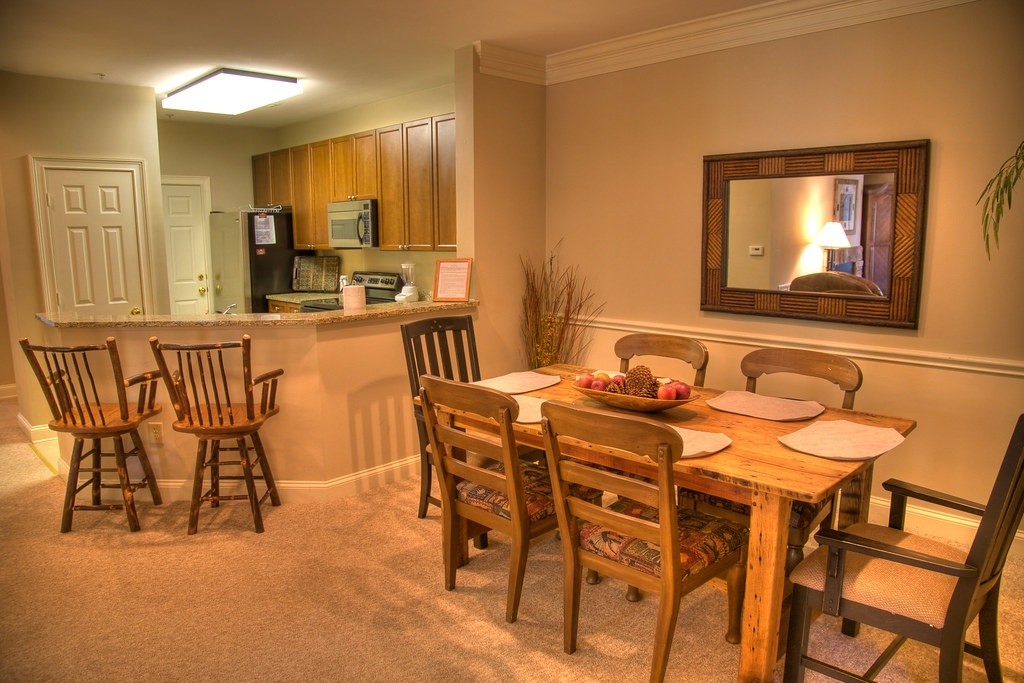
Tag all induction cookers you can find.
[301,270,407,311]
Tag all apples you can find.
[578,372,626,392]
[656,381,691,399]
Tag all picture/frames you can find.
[833,179,859,235]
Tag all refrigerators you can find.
[208,206,315,314]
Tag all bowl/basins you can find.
[571,379,702,411]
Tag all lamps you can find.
[161,67,305,116]
[816,222,852,271]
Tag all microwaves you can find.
[326,199,378,248]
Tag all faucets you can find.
[223,304,238,314]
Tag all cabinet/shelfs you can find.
[268,299,301,313]
[251,112,456,252]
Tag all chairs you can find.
[789,270,884,296]
[789,414,1024,683]
[400,315,864,683]
[149,334,285,534]
[17,336,161,534]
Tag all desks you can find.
[415,362,918,682]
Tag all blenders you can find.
[394,263,418,303]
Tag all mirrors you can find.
[700,139,931,330]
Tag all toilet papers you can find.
[343,284,366,310]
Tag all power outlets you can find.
[147,422,164,444]
